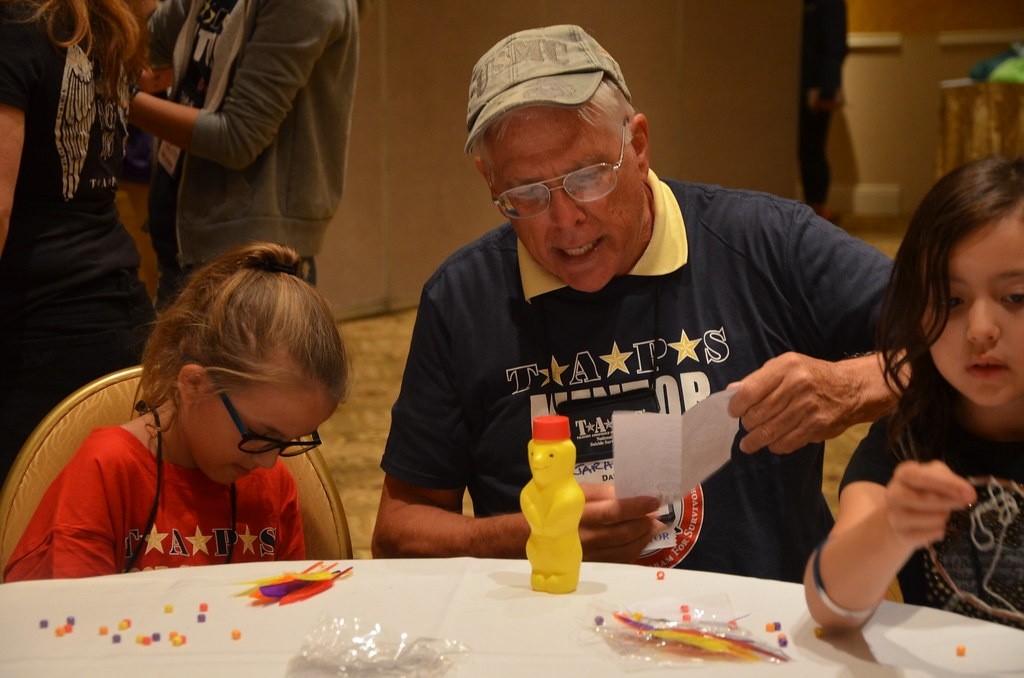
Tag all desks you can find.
[0,557,1024,678]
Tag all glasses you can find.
[181,355,322,457]
[488,116,629,218]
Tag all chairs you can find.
[0,362,353,582]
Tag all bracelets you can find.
[127,85,140,103]
[813,546,874,618]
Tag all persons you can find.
[0,0,1024,678]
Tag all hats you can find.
[463,24,632,156]
